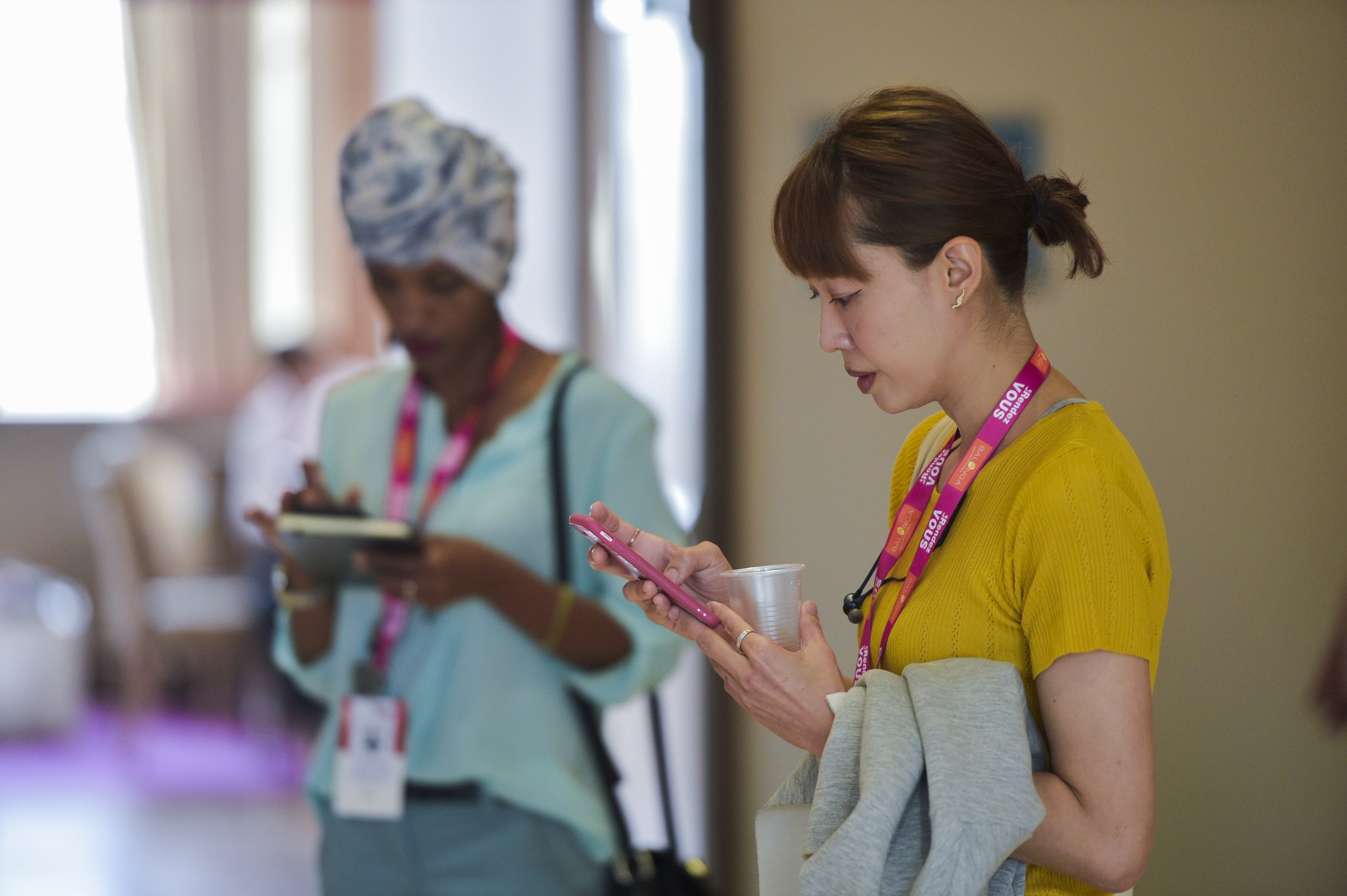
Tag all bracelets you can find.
[276,585,331,611]
[537,582,576,655]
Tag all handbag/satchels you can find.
[602,845,710,896]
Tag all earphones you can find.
[842,592,863,624]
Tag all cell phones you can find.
[569,512,721,629]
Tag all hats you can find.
[338,96,519,295]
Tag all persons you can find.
[223,347,322,712]
[589,84,1172,896]
[243,97,696,896]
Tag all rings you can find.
[736,630,757,654]
[626,527,640,548]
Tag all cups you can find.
[718,562,805,654]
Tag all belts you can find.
[405,782,480,801]
